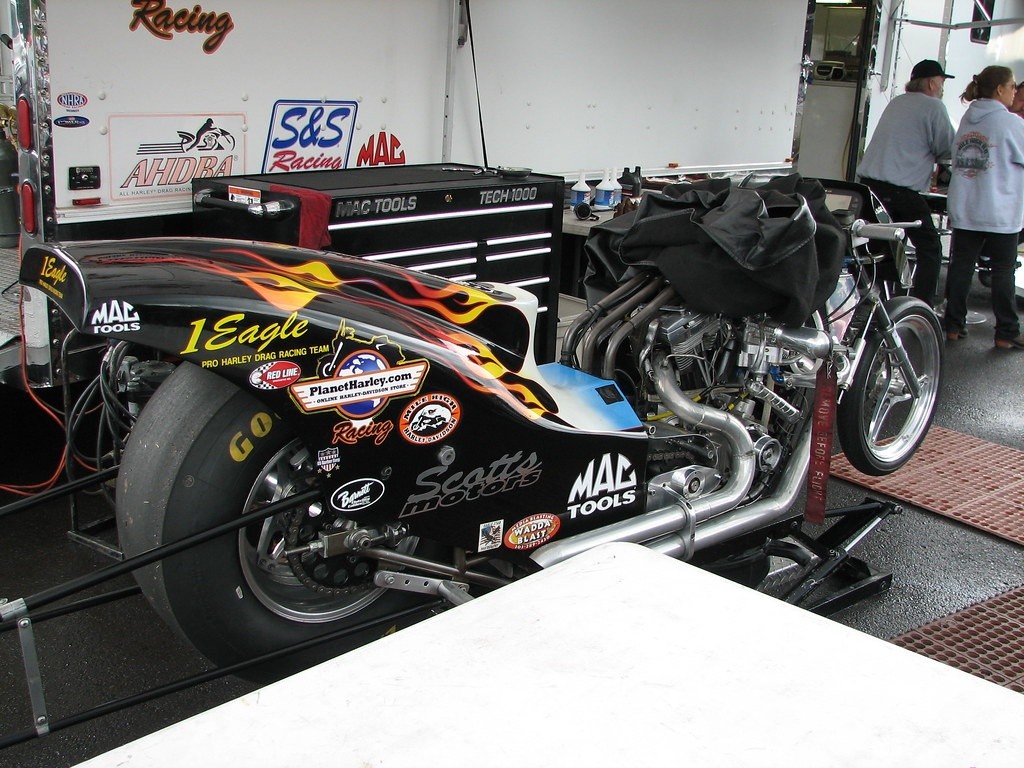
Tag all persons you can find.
[946,66,1024,350]
[856,60,956,329]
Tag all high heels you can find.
[946,326,969,340]
[995,334,1024,349]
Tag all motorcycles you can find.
[0,176,951,752]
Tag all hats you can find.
[910,59,955,82]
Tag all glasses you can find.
[1001,82,1017,90]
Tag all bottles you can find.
[569,170,591,212]
[623,167,631,177]
[594,168,614,210]
[610,167,622,206]
[617,165,642,197]
